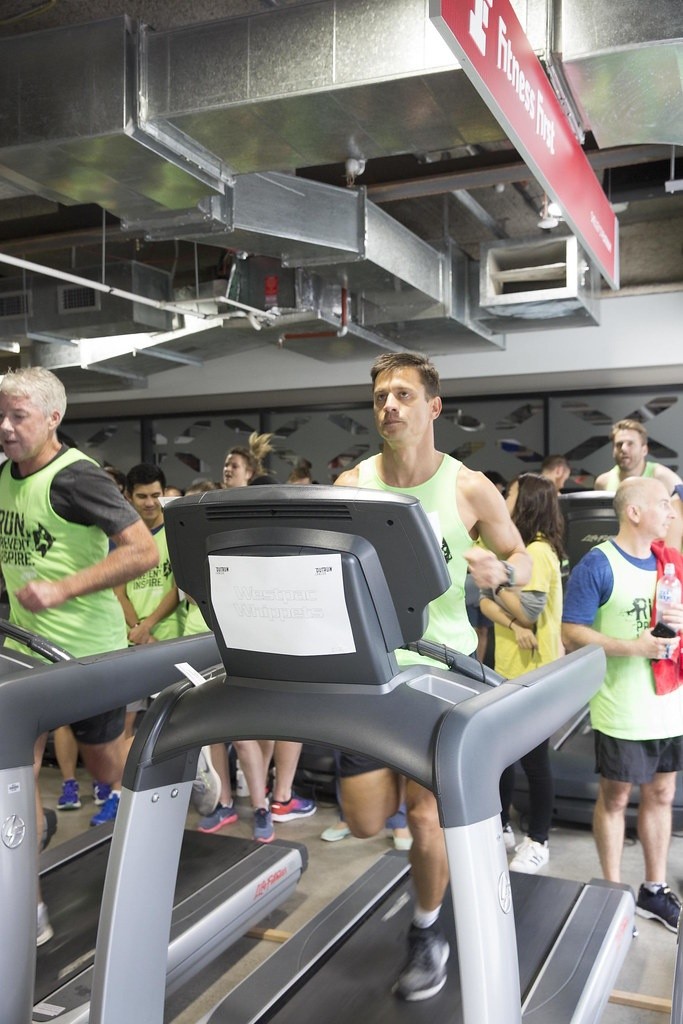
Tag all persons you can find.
[287,467,311,484]
[594,419,683,496]
[0,366,221,946]
[333,352,534,1000]
[223,432,275,488]
[185,477,275,842]
[89,463,187,826]
[541,454,571,495]
[275,754,413,850]
[257,739,317,823]
[561,476,683,938]
[54,725,111,810]
[465,471,564,871]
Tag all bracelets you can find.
[495,585,503,595]
[509,618,516,630]
[501,560,514,587]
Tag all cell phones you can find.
[651,621,676,663]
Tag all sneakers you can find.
[509,837,550,874]
[635,885,682,935]
[503,827,515,849]
[90,793,120,828]
[270,796,318,822]
[198,801,238,834]
[92,781,108,805]
[399,923,450,1001]
[58,781,82,810]
[252,807,275,842]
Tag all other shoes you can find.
[37,905,53,946]
[320,824,352,842]
[392,826,413,850]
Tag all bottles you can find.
[654,563,682,632]
